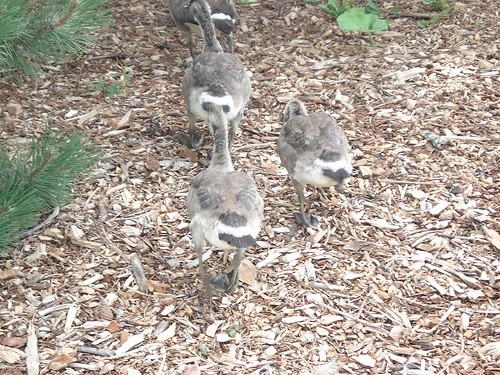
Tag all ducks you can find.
[186,103,264,310]
[167,1,253,151]
[276,98,352,229]
